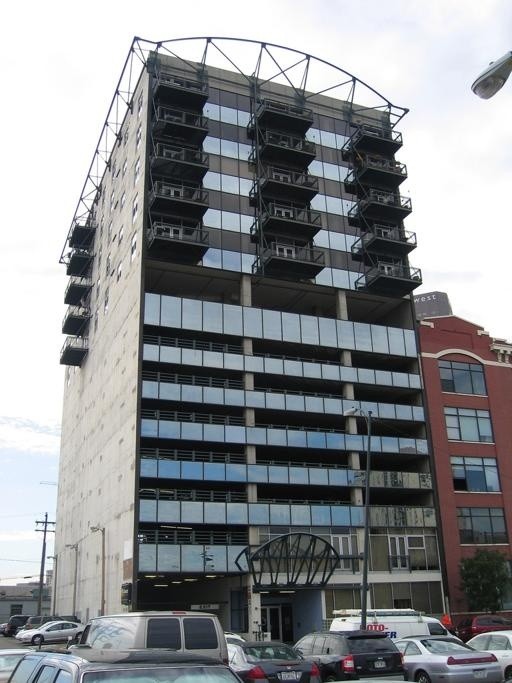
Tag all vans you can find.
[8,647,244,683]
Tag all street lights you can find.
[342,402,373,629]
[66,543,79,618]
[46,554,58,614]
[89,524,105,616]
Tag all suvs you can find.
[458,615,511,643]
[67,609,229,670]
[222,629,246,648]
[1,612,81,636]
[292,629,405,683]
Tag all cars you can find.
[225,641,321,683]
[0,648,37,683]
[13,620,87,646]
[392,634,503,683]
[464,629,511,683]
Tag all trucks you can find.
[328,609,463,645]
